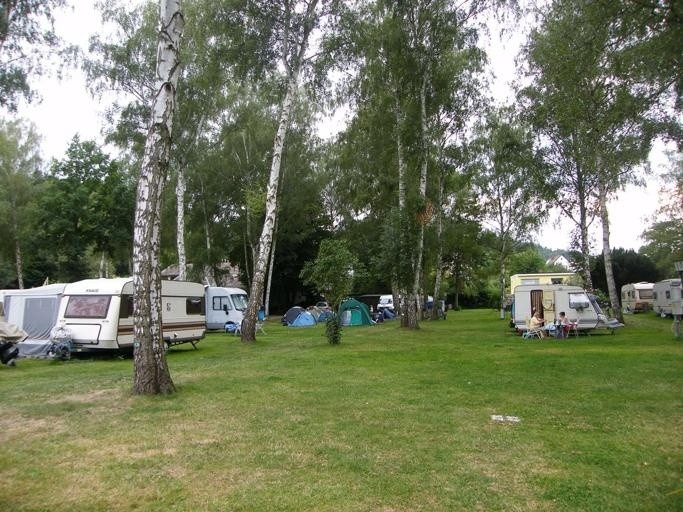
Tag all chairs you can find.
[255,324,267,335]
[224,321,236,334]
[525,316,579,343]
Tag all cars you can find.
[316,301,330,313]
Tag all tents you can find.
[281,298,400,327]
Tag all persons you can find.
[530,311,548,341]
[558,311,572,338]
[46,318,73,360]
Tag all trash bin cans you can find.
[258,310,264,320]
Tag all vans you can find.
[620,280,655,314]
[376,296,404,312]
[206,279,253,333]
[651,277,683,320]
